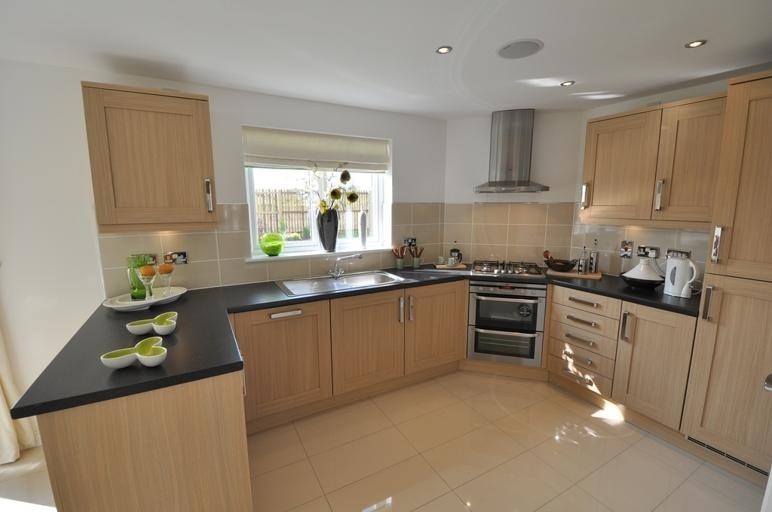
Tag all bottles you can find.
[590,238,599,273]
[578,246,588,274]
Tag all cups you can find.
[136,261,157,299]
[435,257,444,265]
[157,253,177,296]
[413,257,421,268]
[447,257,459,267]
[396,258,405,270]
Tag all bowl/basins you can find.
[619,271,665,292]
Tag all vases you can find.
[317,209,339,252]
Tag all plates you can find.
[103,286,187,313]
[126,311,178,336]
[101,336,167,370]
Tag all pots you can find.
[543,259,578,272]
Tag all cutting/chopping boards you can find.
[433,262,467,270]
[545,267,602,280]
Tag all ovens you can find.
[467,286,546,368]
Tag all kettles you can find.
[664,255,696,298]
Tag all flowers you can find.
[320,170,358,214]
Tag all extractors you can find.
[472,109,549,195]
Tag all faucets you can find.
[329,253,363,279]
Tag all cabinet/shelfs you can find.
[652,93,726,222]
[11,296,254,511]
[81,80,219,232]
[678,275,771,488]
[545,283,622,424]
[704,69,772,281]
[578,109,663,222]
[236,297,333,437]
[334,277,469,410]
[612,301,698,444]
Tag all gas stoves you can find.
[469,258,546,290]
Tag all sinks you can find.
[273,269,405,297]
